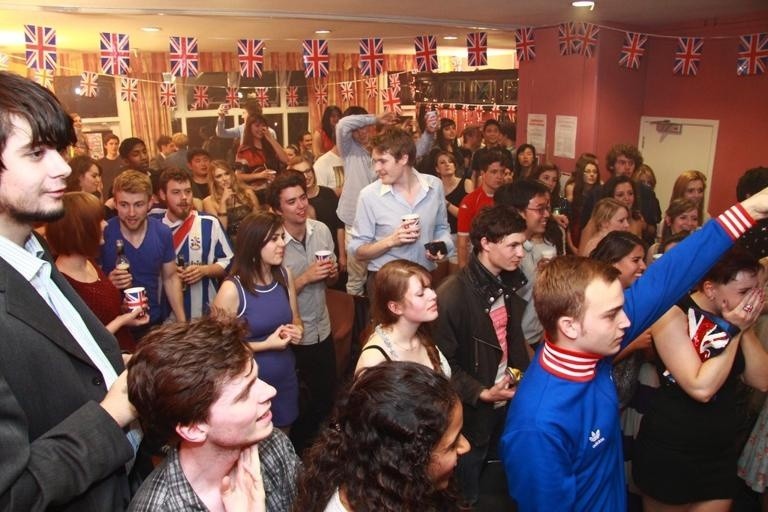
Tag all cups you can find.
[652,252,662,262]
[265,169,277,186]
[314,249,334,267]
[123,286,147,320]
[218,102,229,118]
[400,213,419,232]
[425,111,439,129]
[553,207,563,221]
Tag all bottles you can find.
[114,237,133,293]
[521,239,536,254]
[662,370,676,385]
[177,253,187,292]
[495,366,519,392]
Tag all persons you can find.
[51,98,767,511]
[1,72,142,511]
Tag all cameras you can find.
[222,104,231,110]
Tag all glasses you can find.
[520,205,553,215]
[583,170,598,176]
[302,168,314,175]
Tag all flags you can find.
[24,25,765,115]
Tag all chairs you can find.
[297,287,359,380]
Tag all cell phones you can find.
[424,241,448,254]
